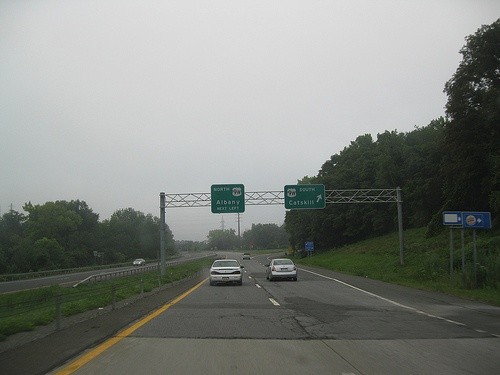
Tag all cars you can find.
[132,258,146,266]
[243,253,251,260]
[265,259,298,281]
[209,259,244,286]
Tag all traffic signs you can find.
[284,184,326,210]
[304,242,314,251]
